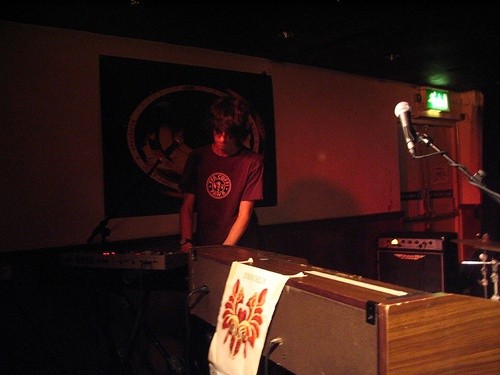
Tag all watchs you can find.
[180,238,191,245]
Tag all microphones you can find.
[394,101,416,156]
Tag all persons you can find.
[178,95,265,255]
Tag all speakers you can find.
[376,232,458,293]
